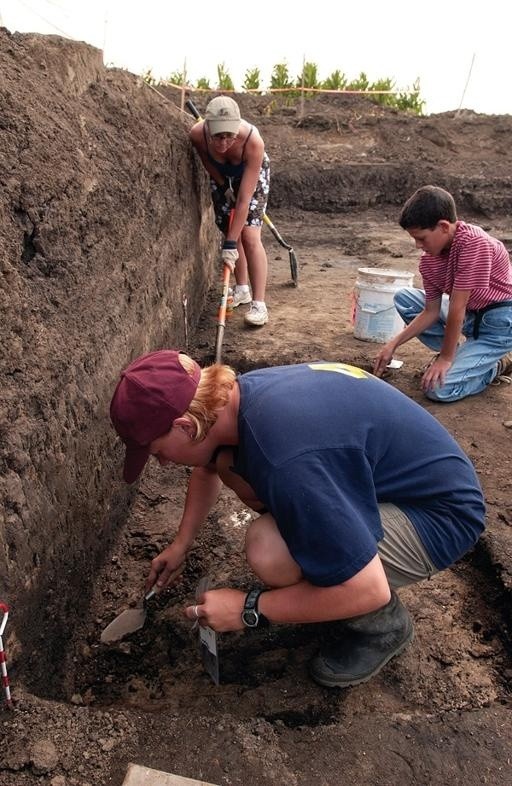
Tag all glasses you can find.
[212,136,238,142]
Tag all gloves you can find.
[219,183,236,203]
[220,239,240,273]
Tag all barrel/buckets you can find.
[353,268,415,343]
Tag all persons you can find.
[187,93,273,329]
[108,350,488,690]
[369,183,511,406]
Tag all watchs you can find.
[240,589,270,630]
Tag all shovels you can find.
[100,560,188,644]
[185,100,299,289]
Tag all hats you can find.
[207,94,241,135]
[111,352,200,484]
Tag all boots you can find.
[311,589,414,689]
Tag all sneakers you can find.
[229,286,252,307]
[244,305,268,326]
[499,352,512,382]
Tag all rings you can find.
[192,605,200,619]
